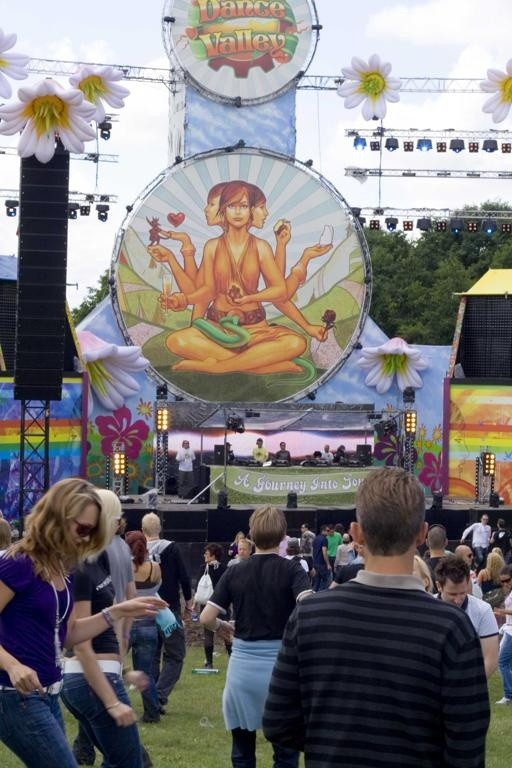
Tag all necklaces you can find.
[41,552,72,666]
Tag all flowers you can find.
[70,65,131,126]
[340,54,403,120]
[482,58,512,123]
[1,77,97,164]
[315,310,336,359]
[75,331,151,421]
[354,335,431,394]
[0,28,33,97]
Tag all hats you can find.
[287,537,299,548]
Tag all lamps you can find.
[342,126,512,158]
[352,207,512,237]
[97,114,112,141]
[83,152,99,163]
[3,189,110,223]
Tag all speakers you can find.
[14,133,69,401]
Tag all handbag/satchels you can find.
[193,572,214,606]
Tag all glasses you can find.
[72,517,96,538]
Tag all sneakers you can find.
[158,700,166,715]
[139,716,160,725]
[496,697,511,705]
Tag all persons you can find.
[311,451,328,466]
[334,445,347,464]
[226,443,235,465]
[175,438,196,496]
[253,438,269,464]
[148,176,340,373]
[274,441,291,463]
[318,444,334,461]
[58,488,144,768]
[300,455,316,466]
[263,465,491,767]
[0,477,170,768]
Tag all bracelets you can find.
[100,608,116,629]
[103,700,123,711]
